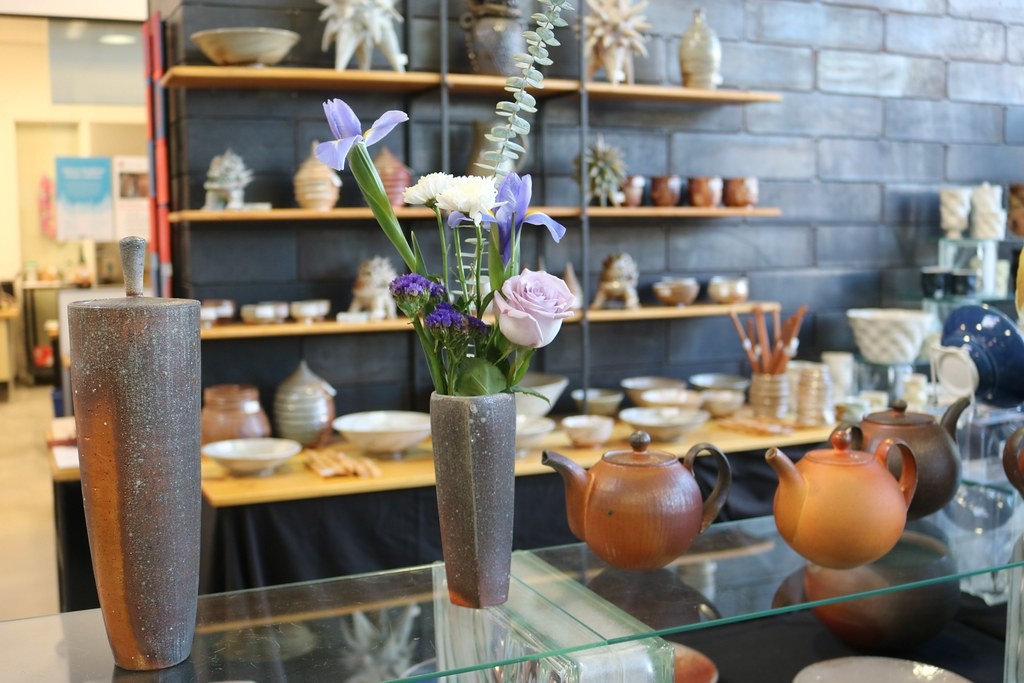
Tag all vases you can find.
[430,390,517,609]
[67,297,200,672]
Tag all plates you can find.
[791,655,975,682]
[662,643,721,683]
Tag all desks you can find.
[201,367,1023,508]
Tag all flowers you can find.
[315,0,576,406]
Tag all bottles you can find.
[676,7,722,92]
[202,383,273,449]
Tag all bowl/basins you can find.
[188,26,303,67]
[846,308,934,365]
[200,435,304,478]
[652,276,750,305]
[498,373,746,454]
[935,304,1024,408]
[331,411,432,455]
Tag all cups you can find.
[609,177,759,206]
[748,367,834,432]
[196,298,331,329]
[821,351,854,394]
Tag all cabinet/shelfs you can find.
[159,62,784,508]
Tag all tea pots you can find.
[829,394,972,523]
[766,426,918,570]
[539,430,731,572]
[801,520,962,646]
